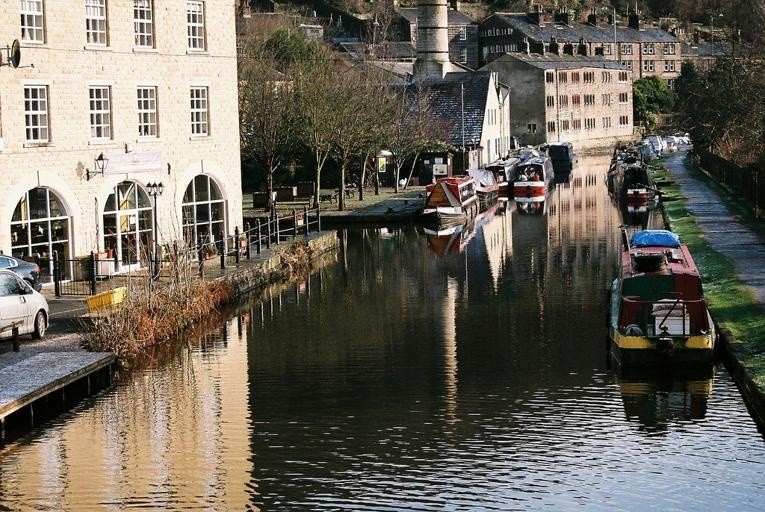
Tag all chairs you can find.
[319,188,355,205]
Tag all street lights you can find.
[710,13,724,70]
[146,181,164,280]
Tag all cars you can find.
[0,254,42,294]
[0,267,49,339]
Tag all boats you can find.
[552,168,575,184]
[496,196,516,218]
[613,198,655,229]
[608,131,692,197]
[484,155,522,200]
[424,176,481,241]
[426,215,480,256]
[609,226,718,368]
[548,142,577,170]
[478,197,503,229]
[513,155,556,197]
[513,190,552,218]
[462,170,499,199]
[613,364,715,431]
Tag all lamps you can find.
[87,152,108,182]
